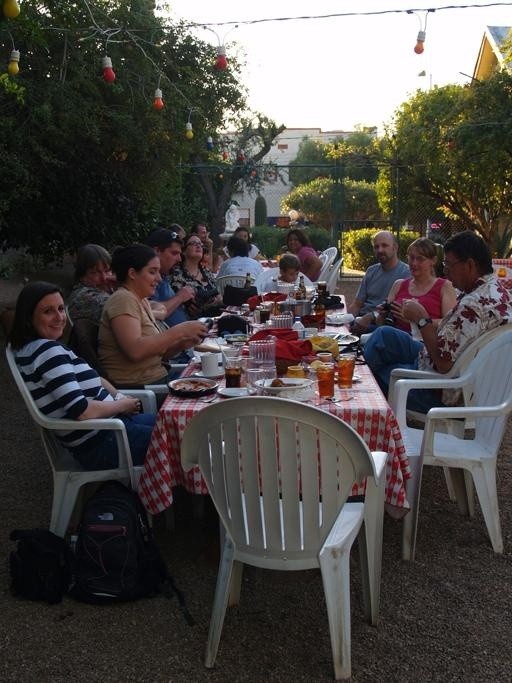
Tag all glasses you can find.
[184,240,204,248]
[171,232,179,239]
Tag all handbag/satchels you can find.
[9,527,75,599]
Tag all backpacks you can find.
[65,487,170,606]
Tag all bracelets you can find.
[113,393,124,401]
[372,311,379,321]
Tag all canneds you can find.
[253,309,260,324]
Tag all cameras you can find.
[382,303,397,326]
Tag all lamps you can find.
[413,29,428,56]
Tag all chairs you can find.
[5,341,157,539]
[388,324,512,562]
[180,396,388,680]
[159,292,392,417]
[318,247,345,295]
[214,275,256,294]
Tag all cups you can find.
[225,338,277,387]
[286,365,306,378]
[199,354,219,373]
[317,281,327,298]
[241,300,273,330]
[317,353,356,399]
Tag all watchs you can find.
[417,318,432,329]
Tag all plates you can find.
[167,375,217,395]
[217,387,256,397]
[193,353,225,366]
[322,332,359,346]
[194,367,227,379]
[326,314,347,324]
[333,373,362,382]
[254,377,312,392]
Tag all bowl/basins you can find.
[276,300,313,317]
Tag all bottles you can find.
[244,272,251,288]
[296,276,306,299]
[292,316,305,341]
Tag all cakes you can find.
[308,335,339,359]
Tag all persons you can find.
[67,244,117,325]
[98,243,208,404]
[363,231,512,421]
[8,281,159,471]
[145,222,323,363]
[347,231,412,333]
[376,238,457,336]
